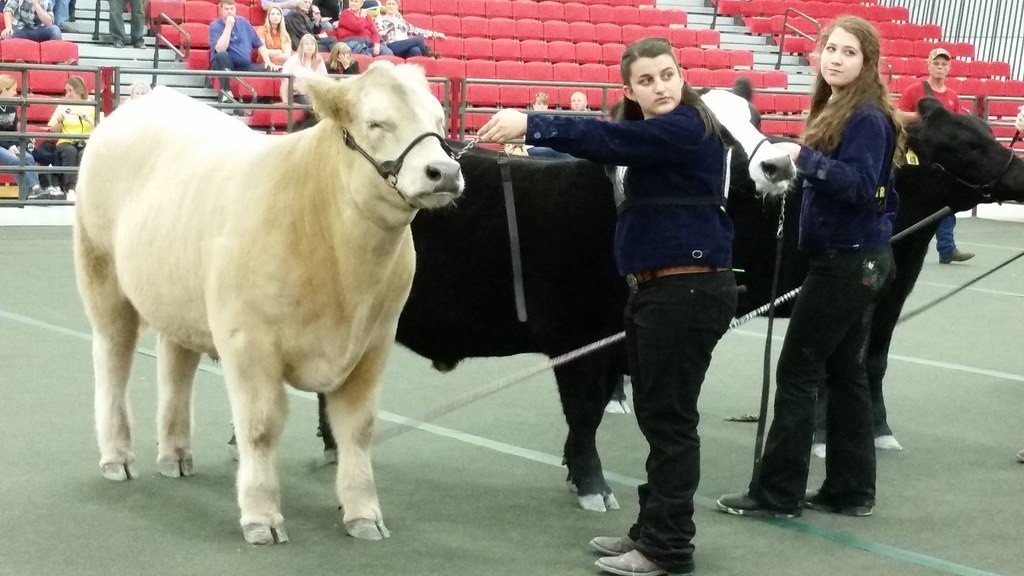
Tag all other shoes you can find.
[222,91,233,103]
[595,543,693,576]
[67,190,76,201]
[134,41,146,49]
[27,188,50,201]
[939,248,974,264]
[589,532,636,555]
[115,41,124,48]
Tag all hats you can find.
[929,48,951,61]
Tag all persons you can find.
[326,43,360,75]
[108,0,146,48]
[718,16,909,520]
[571,91,588,111]
[279,35,327,103]
[533,93,551,110]
[1,75,48,199]
[48,0,71,28]
[475,38,735,576]
[262,0,298,17]
[209,0,282,102]
[1,0,63,41]
[731,79,762,130]
[257,6,292,66]
[374,0,447,56]
[129,81,152,100]
[898,48,975,265]
[49,76,95,200]
[285,0,393,56]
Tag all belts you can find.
[626,264,730,291]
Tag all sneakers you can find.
[805,488,874,517]
[717,486,799,520]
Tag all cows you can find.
[75,62,466,544]
[328,86,808,515]
[604,92,1024,459]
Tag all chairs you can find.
[1,1,1024,184]
